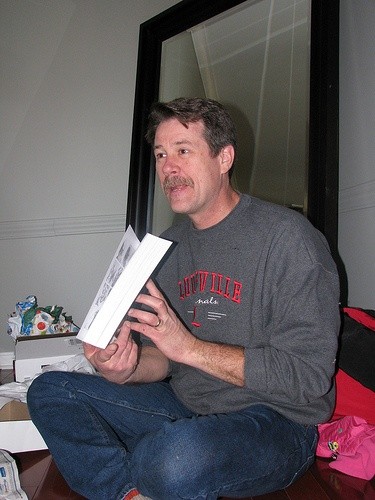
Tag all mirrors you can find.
[125,1,339,256]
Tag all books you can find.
[0,351,15,369]
[75,224,173,350]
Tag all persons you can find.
[26,95,340,500]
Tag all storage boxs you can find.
[14,323,81,384]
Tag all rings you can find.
[153,316,161,327]
